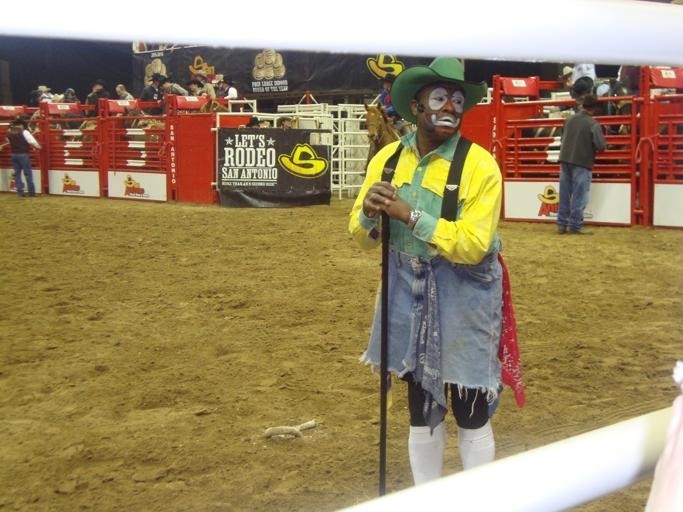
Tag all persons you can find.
[3,116,42,200]
[547,65,637,150]
[346,56,523,486]
[245,116,292,130]
[379,74,413,138]
[553,91,605,234]
[24,72,241,137]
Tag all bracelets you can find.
[407,209,419,229]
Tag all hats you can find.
[557,66,574,80]
[147,70,238,88]
[392,57,487,124]
[570,77,594,98]
